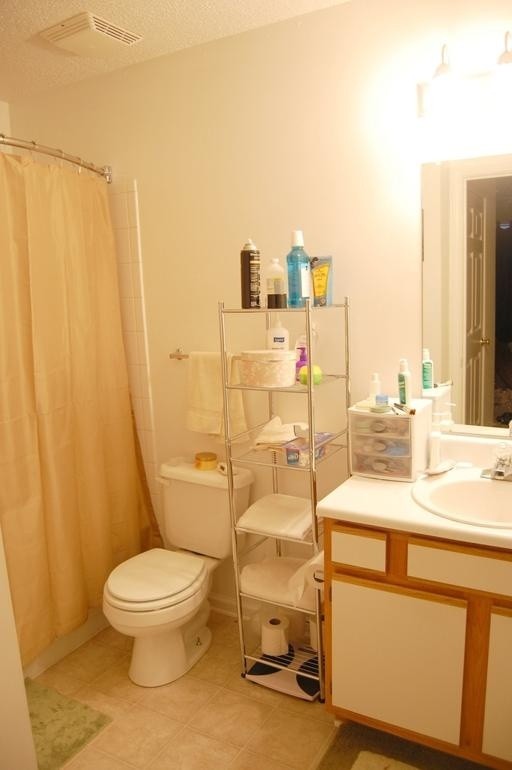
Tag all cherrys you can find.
[432,378,454,388]
[394,403,417,415]
[389,406,399,415]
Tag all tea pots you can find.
[418,154,511,439]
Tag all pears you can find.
[295,347,308,381]
[420,349,433,392]
[286,228,313,304]
[266,311,289,348]
[399,360,410,405]
[266,257,286,307]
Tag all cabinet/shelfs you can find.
[315,454,512,766]
[239,237,262,310]
[286,229,311,308]
[264,257,287,308]
[217,294,351,703]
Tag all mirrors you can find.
[418,154,511,439]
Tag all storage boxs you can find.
[346,396,432,482]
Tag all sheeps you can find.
[492,439,512,479]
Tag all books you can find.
[346,396,432,482]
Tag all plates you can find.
[217,294,351,703]
